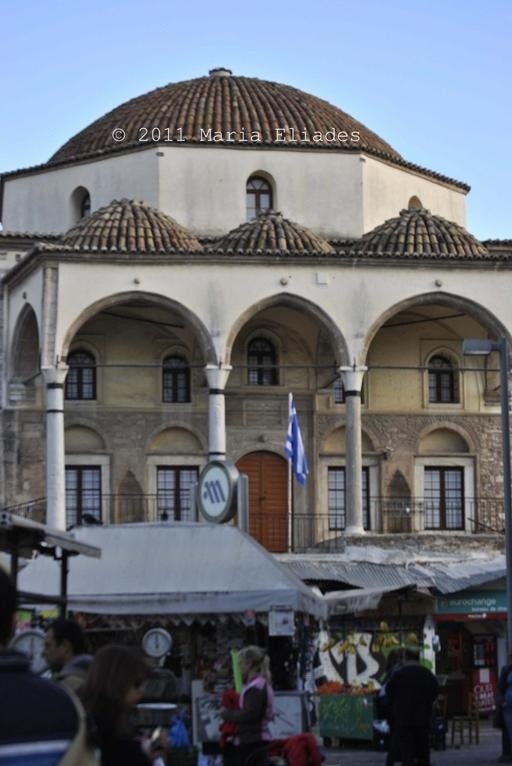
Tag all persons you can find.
[1,568,105,766]
[42,618,101,702]
[490,647,511,766]
[385,646,439,766]
[84,640,172,766]
[212,645,272,766]
[379,646,408,766]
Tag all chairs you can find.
[451,692,480,745]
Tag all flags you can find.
[282,399,310,486]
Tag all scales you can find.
[135,627,180,727]
[6,628,54,678]
